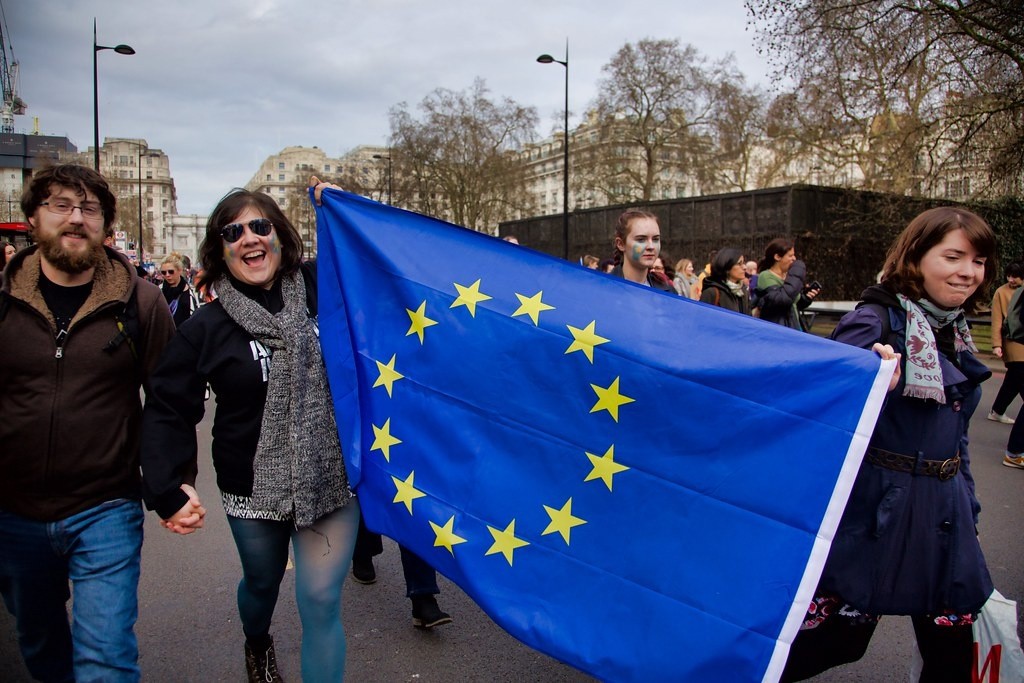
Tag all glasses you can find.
[161,269,177,275]
[733,261,746,266]
[38,200,106,220]
[219,218,273,242]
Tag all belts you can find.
[866,447,959,479]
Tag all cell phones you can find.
[804,280,822,295]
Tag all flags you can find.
[306,184,894,682]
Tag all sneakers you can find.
[243,634,283,683]
[1002,453,1024,468]
[351,557,377,583]
[412,597,454,627]
[987,410,1015,424]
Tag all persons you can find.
[610,212,677,296]
[503,235,519,244]
[649,255,666,274]
[778,208,999,682]
[985,261,1024,470]
[353,516,452,627]
[120,253,217,399]
[143,176,361,683]
[691,252,717,300]
[673,259,695,299]
[698,247,763,318]
[746,261,758,275]
[583,255,614,273]
[758,238,821,332]
[0,242,17,271]
[0,165,204,683]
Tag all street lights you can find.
[535,38,570,261]
[136,140,162,265]
[92,14,137,174]
[373,147,392,206]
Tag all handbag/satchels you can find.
[913,590,1024,683]
